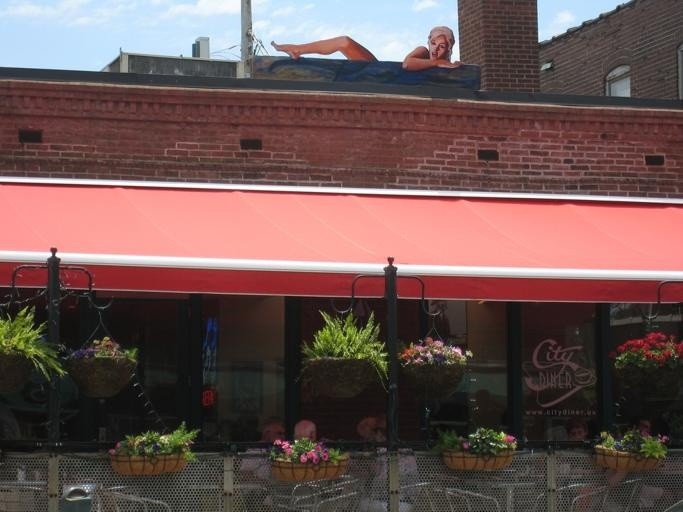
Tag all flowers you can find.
[396,336,474,372]
[429,423,519,459]
[269,436,342,463]
[65,332,136,364]
[609,331,683,371]
[599,422,668,467]
[104,421,199,467]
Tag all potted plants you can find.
[293,309,388,401]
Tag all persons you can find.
[606,416,675,512]
[269,23,467,72]
[238,413,423,511]
[565,419,589,442]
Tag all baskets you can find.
[272,453,350,482]
[64,356,137,400]
[593,445,664,473]
[401,359,464,399]
[620,364,683,403]
[106,449,189,479]
[441,448,513,471]
[309,357,371,399]
[0,355,34,396]
